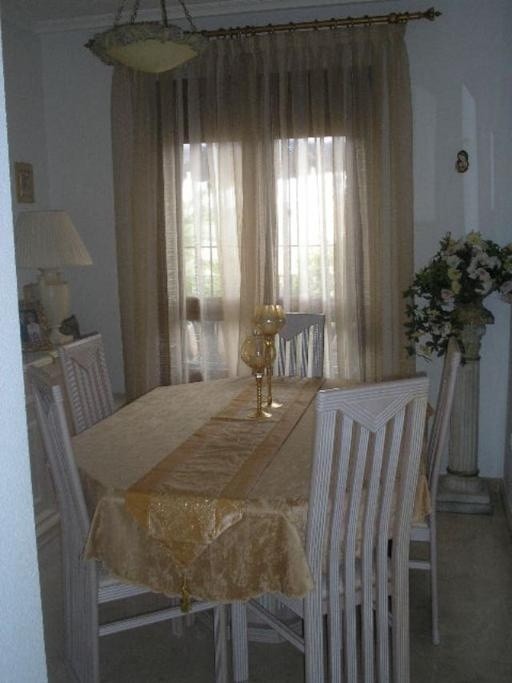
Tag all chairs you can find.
[229,376,432,683]
[408,336,462,642]
[27,363,228,682]
[56,333,117,436]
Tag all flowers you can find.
[401,229,512,361]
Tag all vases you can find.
[436,301,495,517]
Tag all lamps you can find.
[14,209,94,346]
[83,0,209,77]
